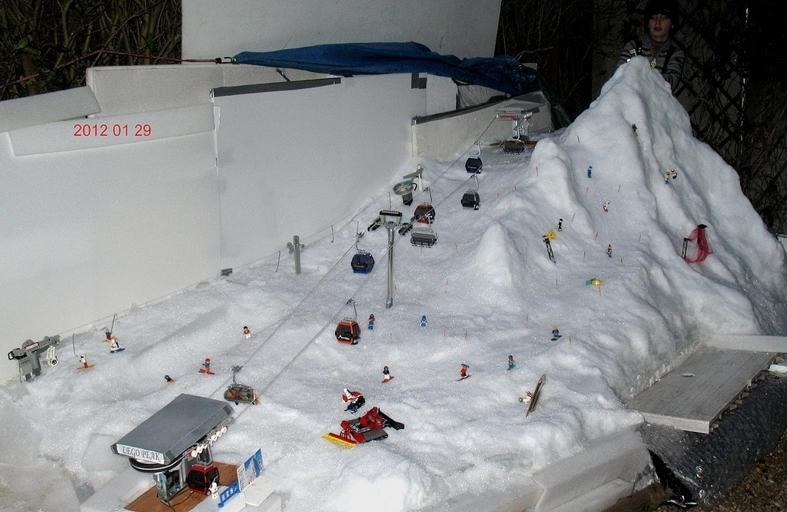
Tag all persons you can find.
[615,0,686,89]
